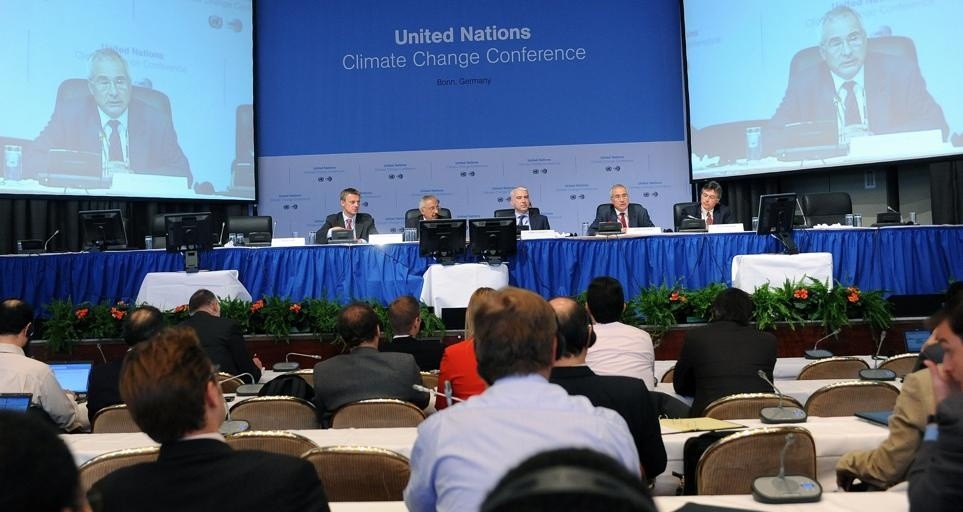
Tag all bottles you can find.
[410,227,418,241]
[581,221,590,236]
[909,211,916,224]
[404,228,410,241]
[844,213,853,225]
[237,233,244,244]
[309,232,317,244]
[752,216,758,231]
[229,233,237,244]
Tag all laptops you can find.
[43,359,91,401]
[1,392,33,415]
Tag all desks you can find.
[2,237,435,311]
[651,492,912,512]
[660,412,905,492]
[516,223,963,306]
[656,378,906,408]
[59,428,419,469]
[654,356,910,383]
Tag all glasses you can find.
[610,194,628,201]
[85,78,131,92]
[817,31,867,51]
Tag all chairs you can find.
[420,370,439,390]
[596,203,640,217]
[796,192,853,228]
[300,444,412,502]
[405,208,451,228]
[148,213,166,249]
[796,356,870,380]
[494,208,539,217]
[215,370,245,393]
[295,369,314,389]
[694,425,817,495]
[224,395,321,430]
[660,366,676,384]
[219,215,272,247]
[804,380,900,417]
[330,397,426,429]
[878,352,919,378]
[91,402,142,433]
[225,429,319,458]
[77,447,160,495]
[702,392,805,420]
[673,202,698,231]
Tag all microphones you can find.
[443,378,454,409]
[217,220,226,245]
[685,213,703,223]
[219,372,256,386]
[757,367,782,411]
[887,205,905,225]
[285,351,322,364]
[873,330,886,374]
[812,328,842,352]
[43,230,61,253]
[796,195,809,226]
[779,432,796,481]
[411,383,466,406]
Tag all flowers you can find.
[827,270,896,330]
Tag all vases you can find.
[847,307,862,319]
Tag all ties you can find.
[842,78,866,126]
[517,214,526,227]
[705,211,712,226]
[346,218,352,231]
[105,119,126,162]
[619,213,627,229]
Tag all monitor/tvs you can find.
[416,218,466,265]
[468,215,517,264]
[756,192,800,255]
[901,327,933,353]
[167,212,217,274]
[76,209,128,253]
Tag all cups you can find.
[229,233,236,244]
[404,228,410,240]
[582,222,589,235]
[410,228,417,241]
[845,214,853,226]
[17,240,22,252]
[910,213,916,224]
[309,232,316,244]
[752,217,758,230]
[292,231,298,238]
[237,234,243,243]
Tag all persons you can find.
[673,289,778,418]
[87,331,331,512]
[403,195,450,242]
[908,295,963,512]
[676,180,736,232]
[587,184,655,235]
[911,309,945,373]
[404,288,641,512]
[435,287,499,410]
[584,276,655,391]
[86,306,164,425]
[316,188,379,244]
[22,48,192,179]
[0,299,80,431]
[174,290,262,385]
[549,299,668,485]
[312,303,430,429]
[388,298,447,371]
[836,280,963,493]
[503,187,550,235]
[0,409,92,512]
[760,5,949,156]
